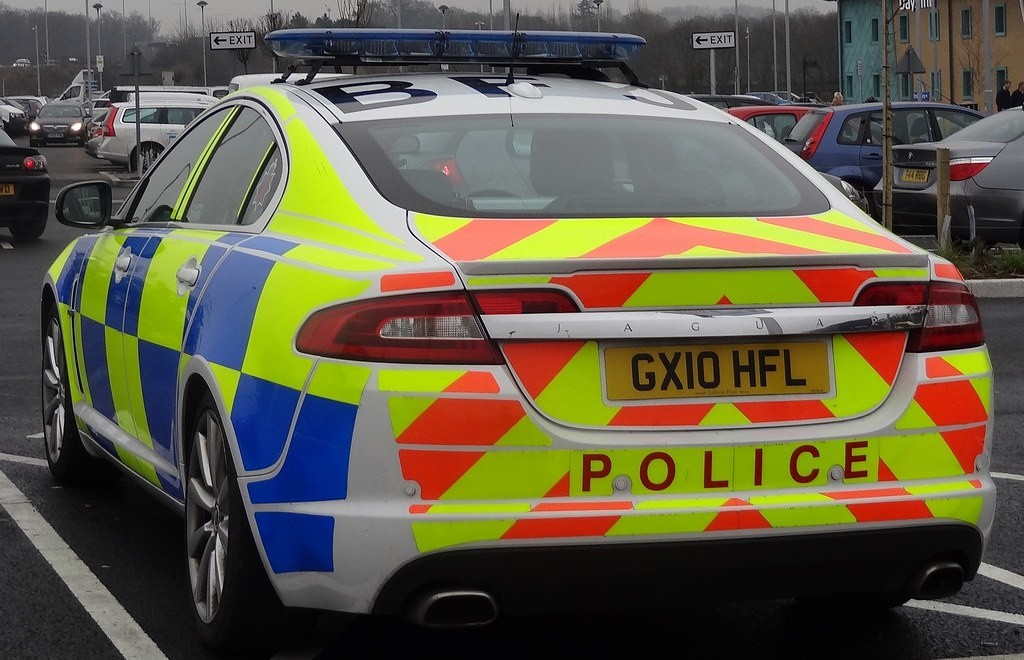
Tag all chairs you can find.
[400,151,732,218]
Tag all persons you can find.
[831,92,846,106]
[1010,82,1024,108]
[995,79,1011,114]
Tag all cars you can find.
[3,20,1024,653]
